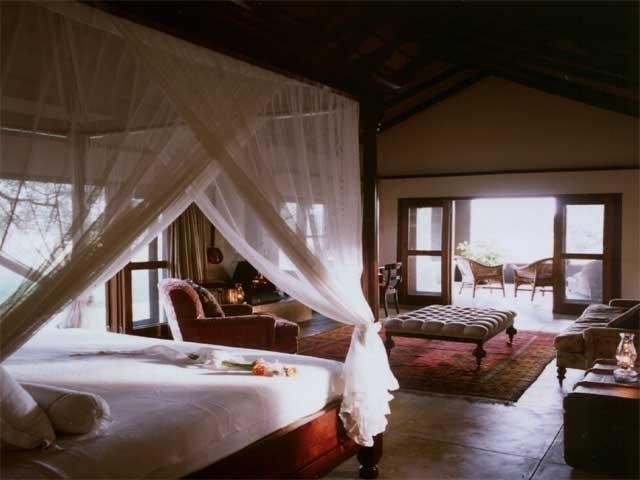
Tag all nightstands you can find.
[382,304,518,368]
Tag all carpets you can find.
[298,316,558,407]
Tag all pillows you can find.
[184,279,227,317]
[0,366,110,455]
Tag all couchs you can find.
[552,298,640,384]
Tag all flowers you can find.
[191,353,273,377]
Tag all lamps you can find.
[613,333,638,383]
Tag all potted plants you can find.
[456,239,500,285]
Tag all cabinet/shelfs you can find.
[561,357,640,476]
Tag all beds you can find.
[0,327,359,480]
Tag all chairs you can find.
[512,257,570,302]
[158,279,301,354]
[379,262,404,317]
[454,256,507,298]
